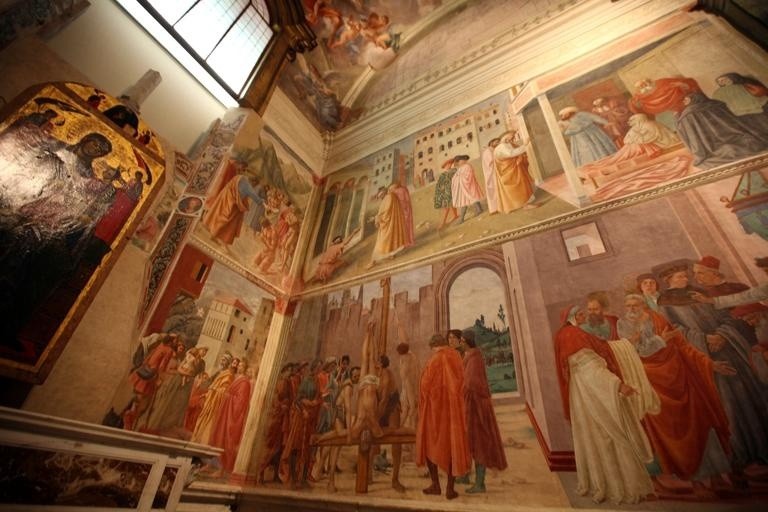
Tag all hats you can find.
[559,106,576,116]
[658,256,719,278]
[280,356,337,371]
[441,154,470,169]
[223,349,233,363]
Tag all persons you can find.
[199,163,280,256]
[390,180,413,246]
[280,0,391,128]
[302,226,363,285]
[365,184,406,269]
[93,169,144,246]
[253,179,302,278]
[0,107,57,137]
[0,132,112,352]
